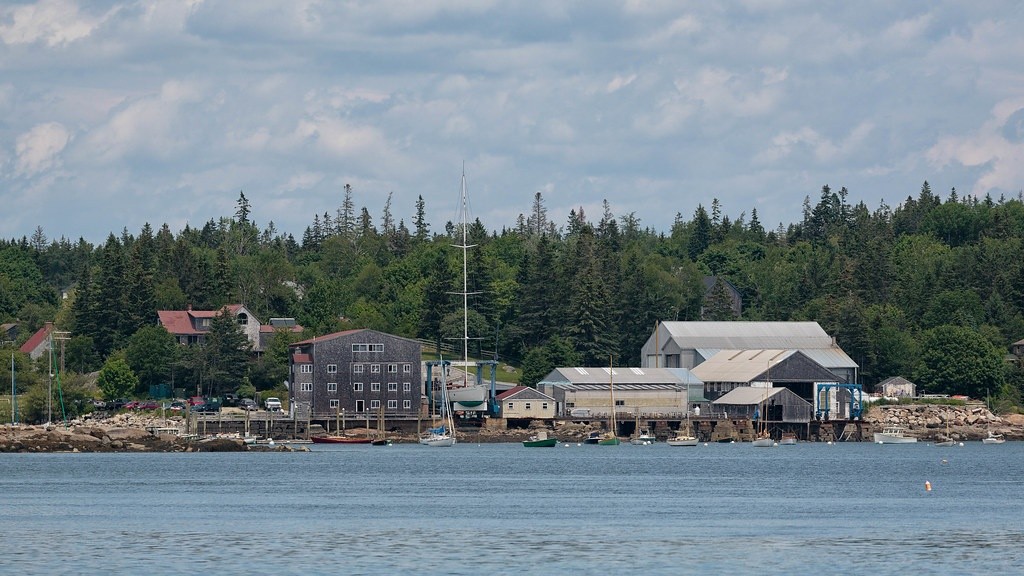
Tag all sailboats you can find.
[419,353,456,446]
[667,383,700,446]
[429,159,488,403]
[936,413,955,447]
[752,362,774,446]
[982,389,1006,445]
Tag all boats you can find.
[523,437,557,447]
[628,433,656,445]
[583,432,621,446]
[371,439,388,445]
[874,427,918,443]
[311,436,374,444]
[780,432,798,445]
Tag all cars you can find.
[85,393,236,415]
[264,398,283,411]
[237,398,259,410]
